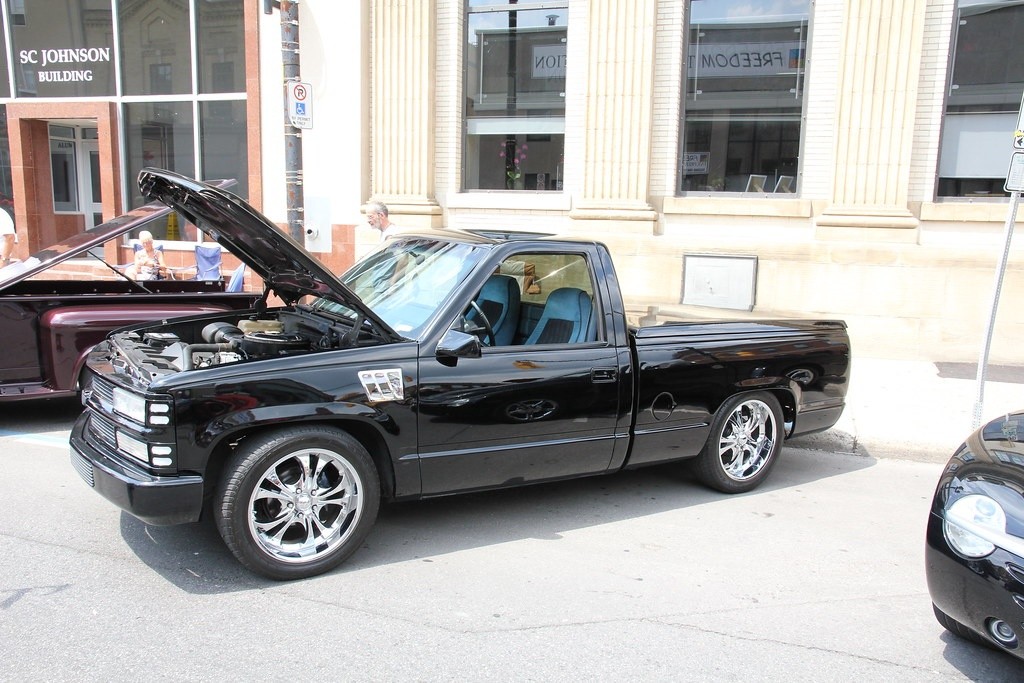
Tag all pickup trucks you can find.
[64,164,853,580]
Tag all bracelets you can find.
[1,256,10,261]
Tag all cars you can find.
[923,410,1023,664]
[1,176,266,417]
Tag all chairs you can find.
[111,244,167,280]
[524,287,598,344]
[464,274,521,345]
[168,245,225,282]
[226,262,246,292]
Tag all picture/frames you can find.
[745,174,767,192]
[773,175,794,193]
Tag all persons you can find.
[366,204,408,303]
[125,231,166,282]
[0,205,19,269]
